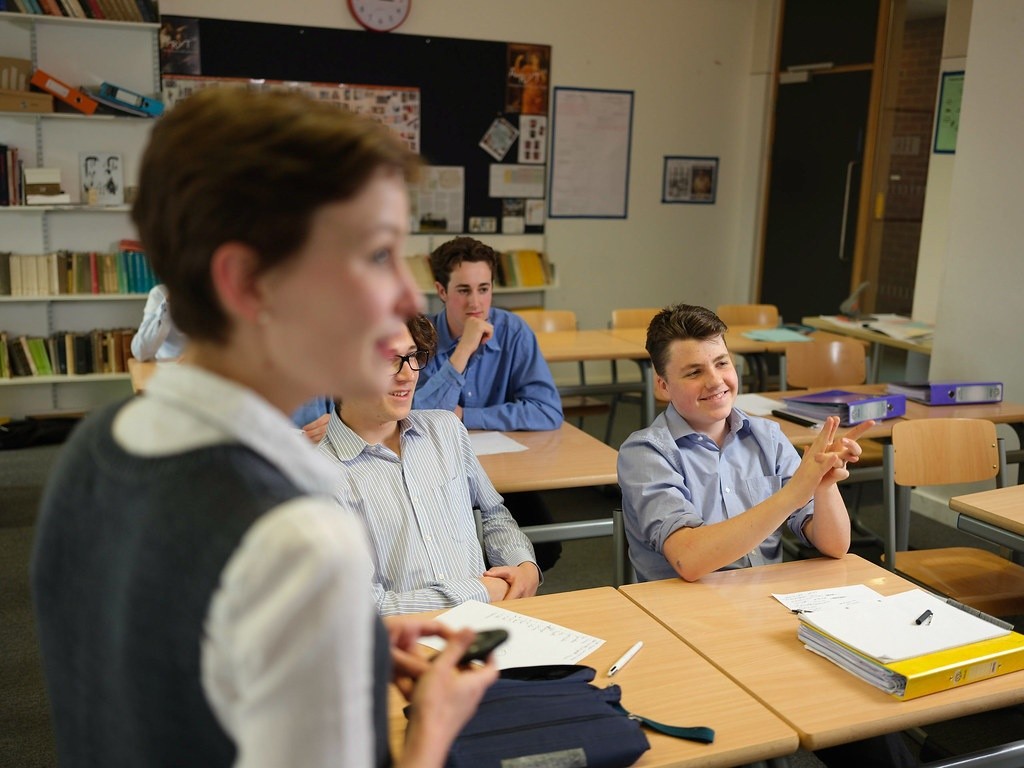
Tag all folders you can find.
[98,82,164,117]
[784,389,905,427]
[798,591,1024,702]
[885,381,1004,407]
[31,69,98,114]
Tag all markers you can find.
[772,409,819,429]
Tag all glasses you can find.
[384,350,429,376]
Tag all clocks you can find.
[347,0,412,32]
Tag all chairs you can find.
[513,304,1023,762]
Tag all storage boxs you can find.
[0,89,54,113]
[24,168,61,195]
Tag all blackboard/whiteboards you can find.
[158,13,553,235]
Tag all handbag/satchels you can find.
[403,662,715,768]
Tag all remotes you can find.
[401,630,508,720]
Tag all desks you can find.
[128,357,157,395]
[619,553,1024,768]
[802,317,932,384]
[386,586,800,768]
[725,324,869,392]
[608,328,767,392]
[535,331,656,428]
[743,391,907,445]
[811,387,1024,486]
[467,420,619,543]
[949,483,1023,554]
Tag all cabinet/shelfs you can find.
[0,11,166,422]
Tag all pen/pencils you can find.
[608,641,642,676]
[792,609,812,614]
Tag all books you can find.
[0,143,124,206]
[0,328,139,379]
[0,0,160,24]
[406,247,548,291]
[0,240,160,296]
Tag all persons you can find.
[130,283,190,362]
[290,395,336,446]
[316,313,544,618]
[28,77,499,767]
[617,304,876,584]
[410,237,564,572]
